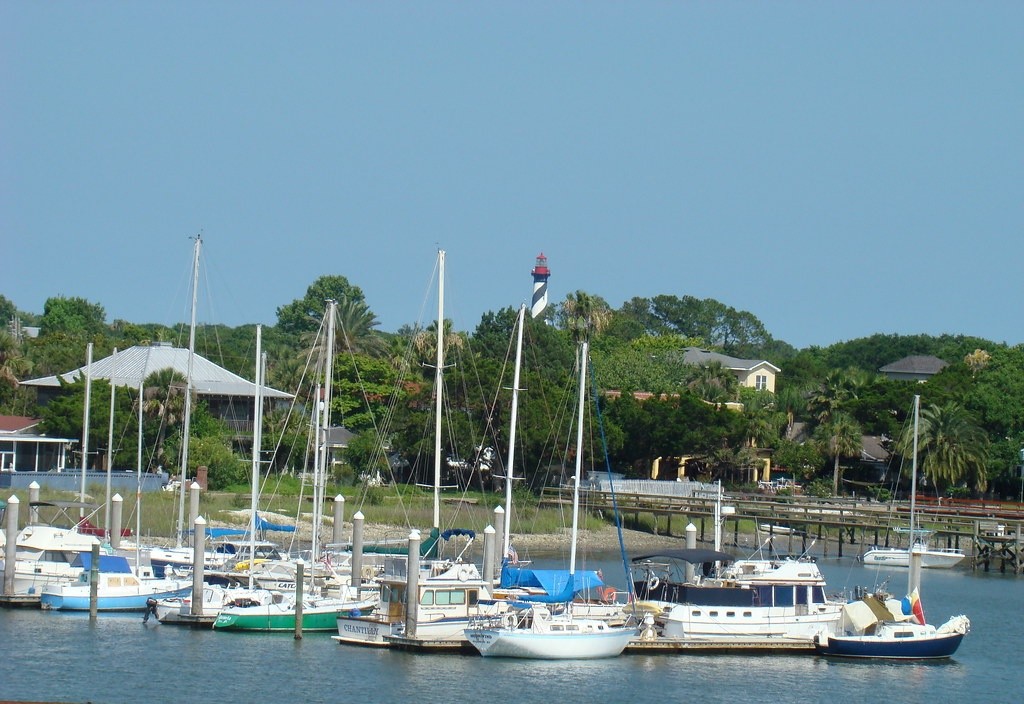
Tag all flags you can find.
[508,546,518,565]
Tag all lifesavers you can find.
[457,568,470,582]
[362,566,375,581]
[603,588,616,601]
[647,576,659,591]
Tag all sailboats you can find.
[811,391,971,659]
[0,222,868,661]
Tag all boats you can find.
[856,506,966,569]
